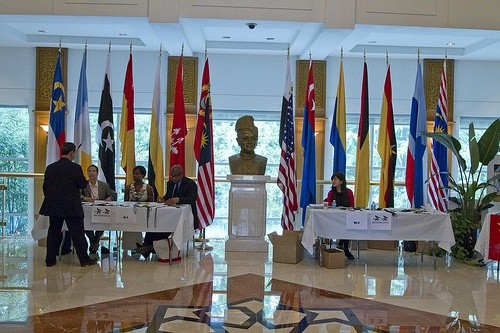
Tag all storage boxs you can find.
[321,247,346,270]
[366,238,397,251]
[267,229,303,264]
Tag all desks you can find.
[300,205,456,270]
[30,199,195,266]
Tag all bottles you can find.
[332,200,336,209]
[371,201,376,210]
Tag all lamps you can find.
[39,124,48,149]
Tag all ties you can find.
[173,183,178,197]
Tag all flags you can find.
[377,65,397,207]
[276,55,300,231]
[330,59,347,177]
[405,61,428,208]
[353,62,370,208]
[74,51,92,181]
[148,54,164,202]
[299,57,316,228]
[96,50,116,191]
[169,56,188,177]
[428,60,452,212]
[193,58,216,231]
[119,52,137,187]
[45,50,67,169]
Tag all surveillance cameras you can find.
[248,24,255,29]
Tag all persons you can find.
[79,164,115,254]
[135,165,199,259]
[122,166,153,253]
[324,171,355,260]
[229,115,268,175]
[39,142,98,268]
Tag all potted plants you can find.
[424,117,500,267]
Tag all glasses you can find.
[170,173,181,178]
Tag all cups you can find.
[323,202,329,209]
[425,203,430,212]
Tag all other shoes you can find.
[136,242,150,258]
[47,260,57,266]
[89,238,99,252]
[345,250,354,260]
[80,259,97,266]
[61,249,71,254]
